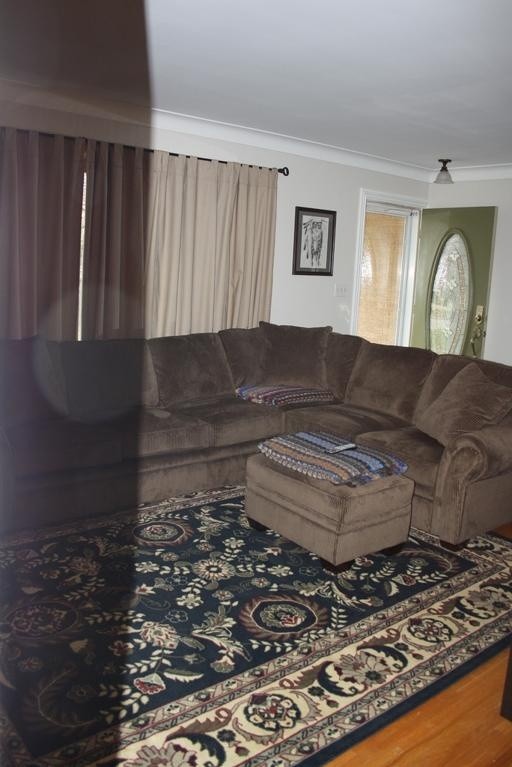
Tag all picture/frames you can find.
[292,206,337,277]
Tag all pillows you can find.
[258,432,407,487]
[0,322,512,448]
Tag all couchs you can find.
[0,322,512,546]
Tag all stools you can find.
[247,454,415,574]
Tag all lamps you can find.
[433,158,454,185]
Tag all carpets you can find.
[0,487,512,765]
